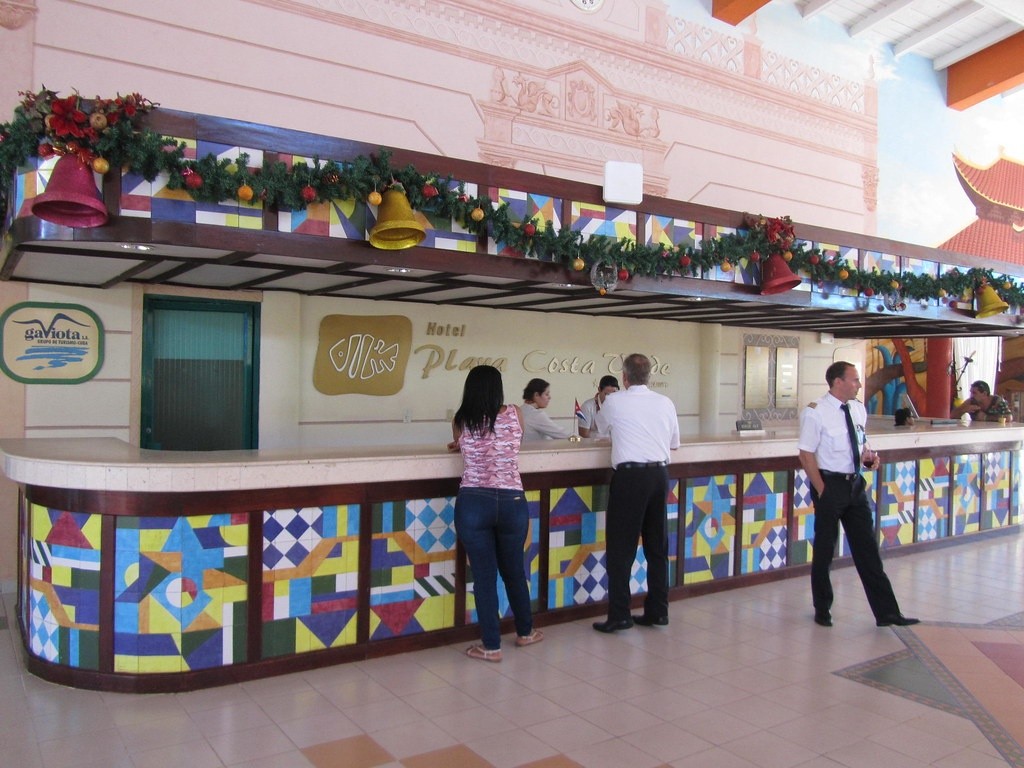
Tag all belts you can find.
[818,469,857,481]
[617,462,667,470]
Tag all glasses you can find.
[909,415,916,418]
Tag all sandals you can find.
[465,643,502,663]
[516,626,544,645]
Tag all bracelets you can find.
[983,414,988,421]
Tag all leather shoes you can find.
[632,613,668,626]
[876,611,920,627]
[813,608,832,626]
[592,617,633,633]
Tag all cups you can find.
[862,450,878,469]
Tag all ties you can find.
[840,403,860,476]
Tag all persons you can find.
[893,407,914,426]
[577,376,620,438]
[949,380,1013,422]
[798,361,929,633]
[447,365,544,662]
[592,354,681,632]
[518,378,572,444]
